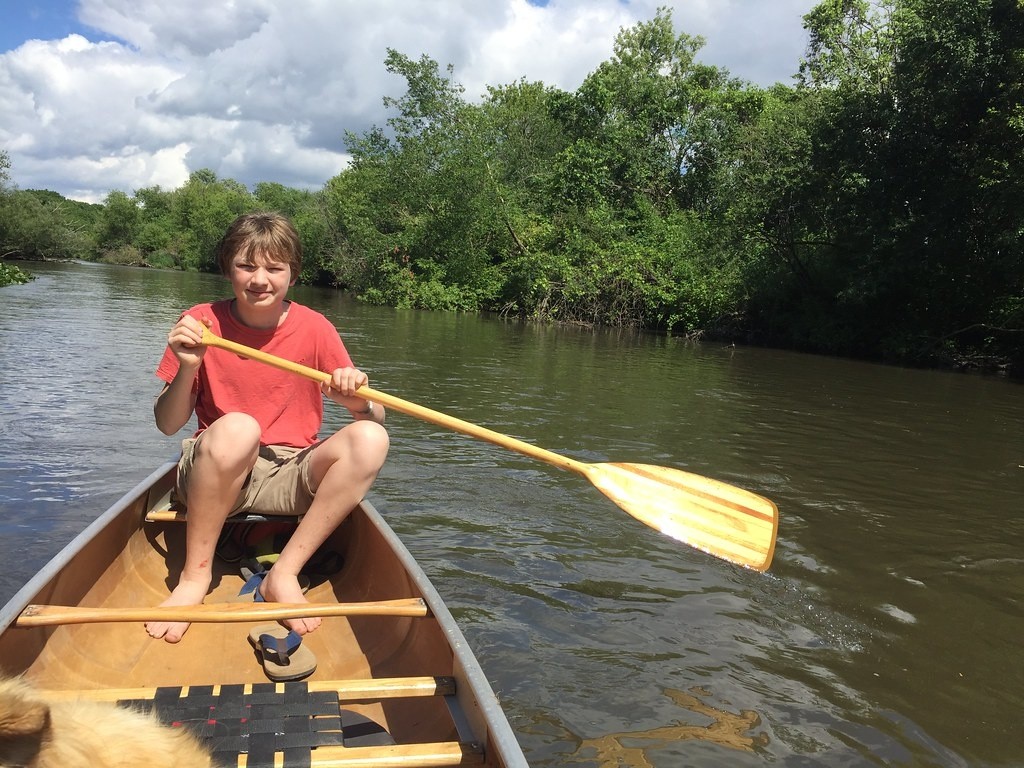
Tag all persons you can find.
[142,212,390,643]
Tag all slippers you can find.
[225,568,310,604]
[250,623,318,682]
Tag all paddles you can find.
[197,328,778,569]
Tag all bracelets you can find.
[354,400,373,414]
